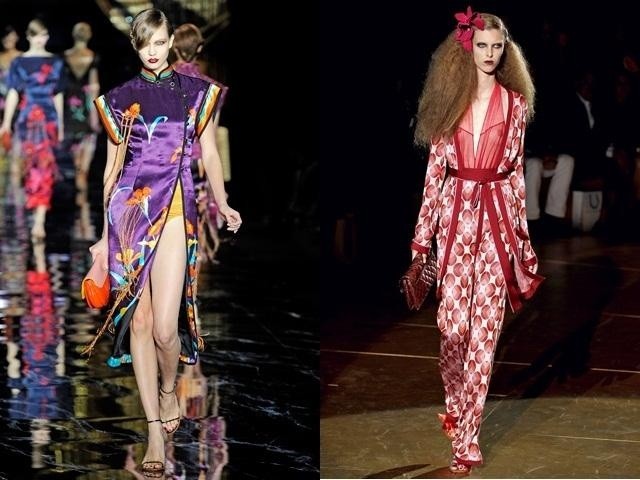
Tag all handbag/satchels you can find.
[81,254,111,309]
[571,192,603,233]
[398,249,436,311]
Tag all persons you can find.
[400,7,545,471]
[565,69,627,230]
[0,30,23,107]
[524,137,574,238]
[171,24,230,355]
[1,21,67,243]
[17,244,67,445]
[82,8,243,473]
[63,21,98,193]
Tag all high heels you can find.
[141,383,181,473]
